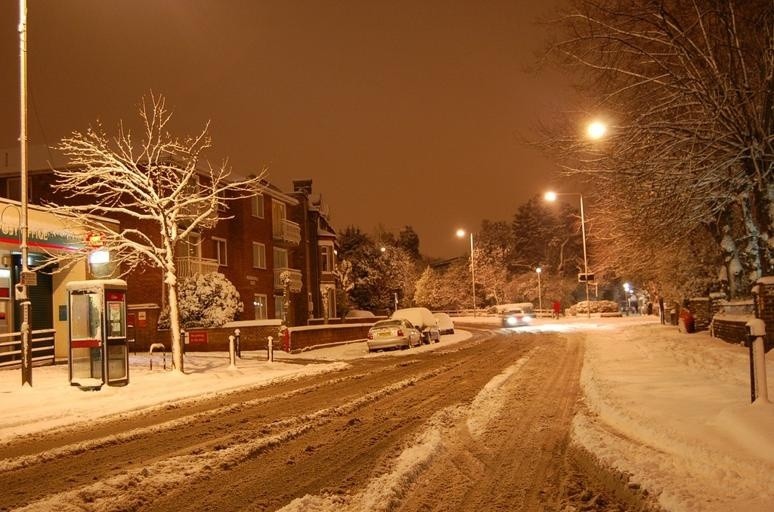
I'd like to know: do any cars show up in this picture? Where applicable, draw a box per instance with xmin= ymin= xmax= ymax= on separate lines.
xmin=366 ymin=308 xmax=454 ymax=353
xmin=501 ymin=308 xmax=530 ymax=327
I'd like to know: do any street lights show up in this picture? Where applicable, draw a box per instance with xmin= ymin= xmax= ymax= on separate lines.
xmin=535 ymin=267 xmax=543 ymax=320
xmin=623 ymin=283 xmax=633 ymax=317
xmin=541 ymin=190 xmax=591 ymax=318
xmin=455 ymin=227 xmax=476 ymax=318
xmin=380 ymin=246 xmax=397 ymax=310
